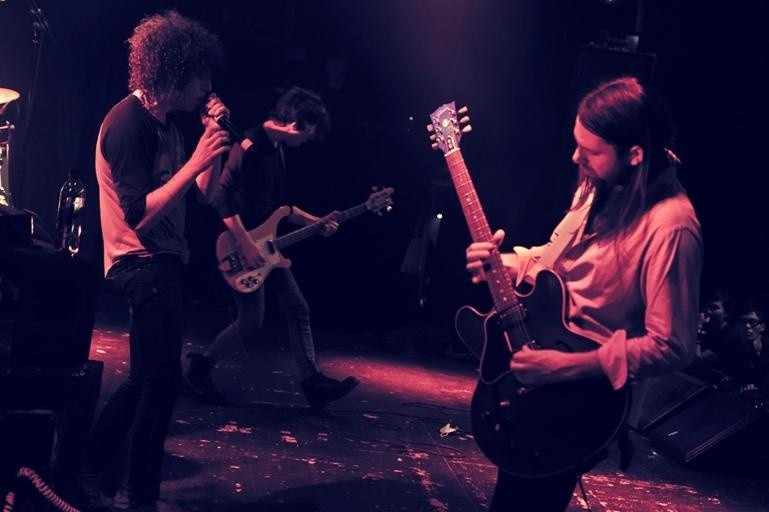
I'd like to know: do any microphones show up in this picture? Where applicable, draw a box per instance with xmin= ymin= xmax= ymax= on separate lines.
xmin=200 ymin=95 xmax=255 ymax=152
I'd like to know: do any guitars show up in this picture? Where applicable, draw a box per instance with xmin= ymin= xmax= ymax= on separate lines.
xmin=427 ymin=101 xmax=632 ymax=478
xmin=216 ymin=185 xmax=394 ymax=292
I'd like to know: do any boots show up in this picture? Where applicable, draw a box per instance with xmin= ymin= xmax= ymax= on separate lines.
xmin=182 ymin=352 xmax=227 ymax=404
xmin=299 ymin=370 xmax=359 ymax=411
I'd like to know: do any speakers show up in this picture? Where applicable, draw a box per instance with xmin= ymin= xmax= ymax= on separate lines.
xmin=624 ymin=362 xmax=714 ymax=436
xmin=650 ymin=387 xmax=769 ymax=470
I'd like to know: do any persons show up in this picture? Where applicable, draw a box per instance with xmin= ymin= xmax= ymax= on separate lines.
xmin=690 ymin=288 xmax=741 ymax=378
xmin=175 ymin=84 xmax=360 ymax=410
xmin=731 ymin=296 xmax=768 ymax=414
xmin=464 ymin=74 xmax=704 ymax=511
xmin=84 ymin=11 xmax=230 ymax=509
